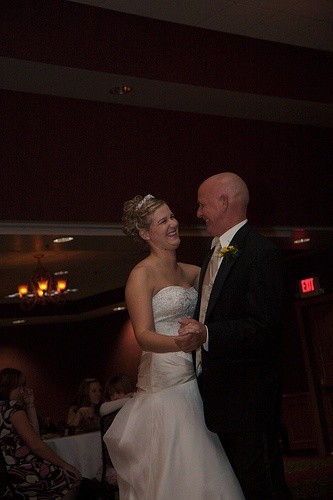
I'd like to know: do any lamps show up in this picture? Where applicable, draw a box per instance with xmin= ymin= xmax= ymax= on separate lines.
xmin=17 ymin=254 xmax=81 ymax=310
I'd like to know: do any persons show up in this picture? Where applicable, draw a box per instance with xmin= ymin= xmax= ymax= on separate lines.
xmin=67 ymin=378 xmax=105 ymax=433
xmin=0 ymin=368 xmax=82 ymax=500
xmin=99 ymin=375 xmax=134 ymax=486
xmin=102 ymin=194 xmax=245 ymax=500
xmin=23 ymin=387 xmax=40 ymax=437
xmin=179 ymin=172 xmax=292 ymax=500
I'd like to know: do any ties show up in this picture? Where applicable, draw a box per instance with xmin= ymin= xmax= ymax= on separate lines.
xmin=195 ymin=241 xmax=222 ymax=376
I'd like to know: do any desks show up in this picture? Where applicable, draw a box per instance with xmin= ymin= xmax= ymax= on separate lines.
xmin=41 ymin=430 xmax=103 ymax=481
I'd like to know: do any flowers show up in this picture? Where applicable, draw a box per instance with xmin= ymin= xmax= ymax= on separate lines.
xmin=216 ymin=240 xmax=239 ymax=263
